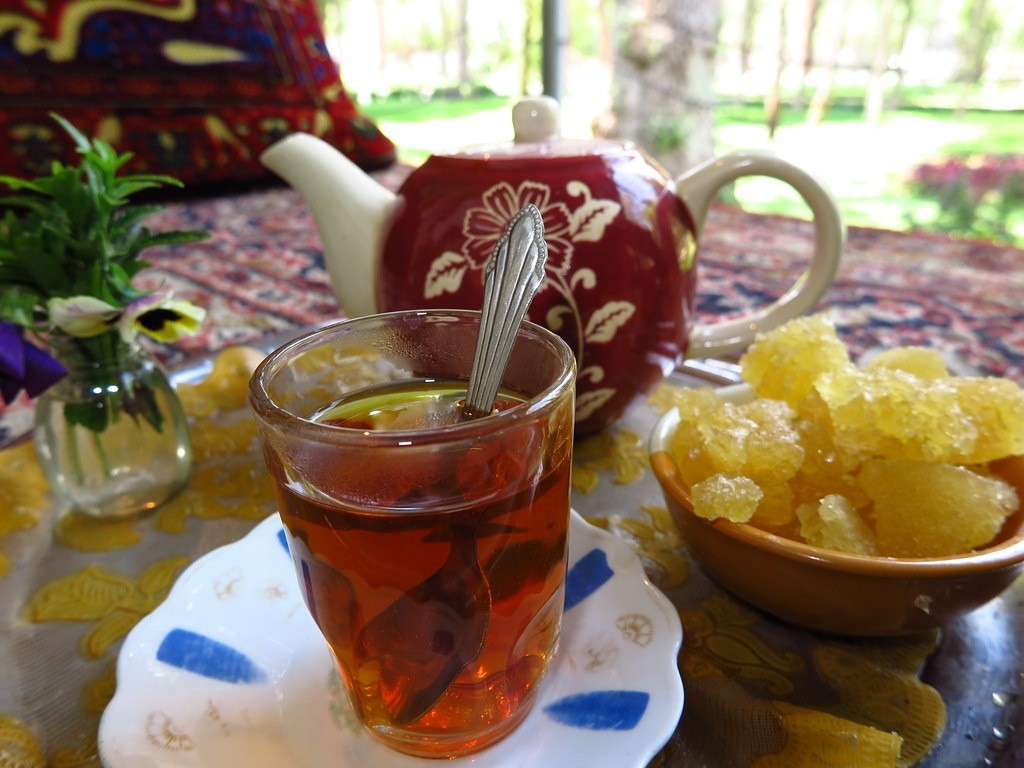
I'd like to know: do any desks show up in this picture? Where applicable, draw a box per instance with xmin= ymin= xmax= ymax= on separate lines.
xmin=0 ymin=317 xmax=1023 ymax=766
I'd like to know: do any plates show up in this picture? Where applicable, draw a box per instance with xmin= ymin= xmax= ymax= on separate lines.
xmin=96 ymin=504 xmax=687 ymax=767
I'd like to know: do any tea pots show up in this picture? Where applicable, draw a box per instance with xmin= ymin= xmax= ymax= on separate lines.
xmin=258 ymin=94 xmax=848 ymax=445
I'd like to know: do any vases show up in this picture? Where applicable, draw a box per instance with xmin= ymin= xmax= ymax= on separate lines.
xmin=32 ymin=326 xmax=193 ymax=520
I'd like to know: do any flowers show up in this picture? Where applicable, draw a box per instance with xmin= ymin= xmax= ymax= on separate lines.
xmin=0 ymin=111 xmax=213 ymax=485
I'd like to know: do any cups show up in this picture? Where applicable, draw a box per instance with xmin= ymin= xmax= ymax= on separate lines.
xmin=245 ymin=309 xmax=577 ymax=758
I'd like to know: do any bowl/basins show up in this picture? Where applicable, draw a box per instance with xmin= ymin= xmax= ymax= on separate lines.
xmin=646 ymin=382 xmax=1024 ymax=637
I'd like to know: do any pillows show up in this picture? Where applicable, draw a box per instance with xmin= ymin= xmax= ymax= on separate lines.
xmin=0 ymin=0 xmax=395 ymax=201
